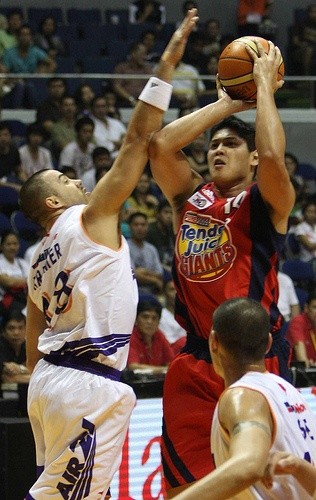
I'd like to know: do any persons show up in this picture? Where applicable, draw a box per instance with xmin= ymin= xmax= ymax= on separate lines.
xmin=0 ymin=0 xmax=316 ymax=500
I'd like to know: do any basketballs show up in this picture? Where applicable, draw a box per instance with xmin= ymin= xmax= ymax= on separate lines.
xmin=217 ymin=35 xmax=285 ymax=104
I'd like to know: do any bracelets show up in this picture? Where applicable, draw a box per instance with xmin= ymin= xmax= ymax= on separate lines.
xmin=137 ymin=77 xmax=174 ymax=114
